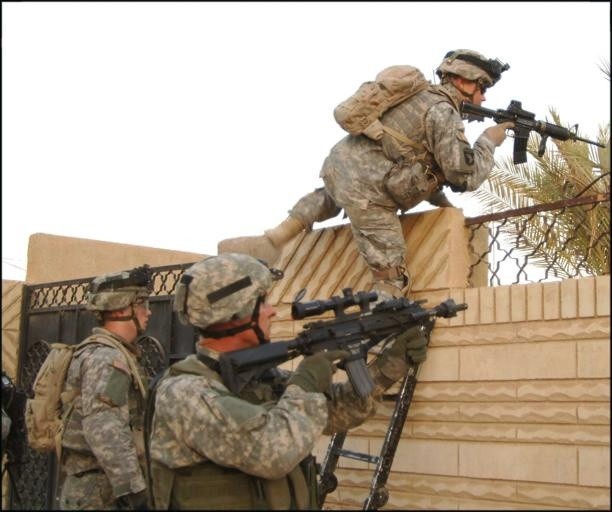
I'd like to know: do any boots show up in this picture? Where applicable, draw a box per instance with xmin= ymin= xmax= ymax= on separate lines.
xmin=263 ymin=216 xmax=306 ymax=248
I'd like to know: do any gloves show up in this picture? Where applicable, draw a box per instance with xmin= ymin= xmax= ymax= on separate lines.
xmin=367 ymin=325 xmax=430 ymax=388
xmin=286 ymin=348 xmax=353 ymax=395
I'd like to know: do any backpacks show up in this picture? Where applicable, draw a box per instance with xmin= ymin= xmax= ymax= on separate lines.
xmin=23 ymin=335 xmax=149 ymax=451
xmin=332 ymin=64 xmax=459 ymax=151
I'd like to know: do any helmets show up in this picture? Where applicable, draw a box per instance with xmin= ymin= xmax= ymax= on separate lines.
xmin=435 ymin=48 xmax=510 ymax=89
xmin=82 ymin=264 xmax=155 ymax=313
xmin=170 ymin=253 xmax=284 ymax=328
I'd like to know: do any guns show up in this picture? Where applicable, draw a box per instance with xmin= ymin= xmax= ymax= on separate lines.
xmin=460 ymin=100 xmax=607 ymax=164
xmin=218 ymin=288 xmax=469 ymax=397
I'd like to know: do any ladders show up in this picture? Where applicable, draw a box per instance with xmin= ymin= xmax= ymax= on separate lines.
xmin=318 ymin=314 xmax=436 ymax=512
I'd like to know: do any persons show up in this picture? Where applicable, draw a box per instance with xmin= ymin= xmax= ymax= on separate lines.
xmin=142 ymin=251 xmax=430 ymax=510
xmin=56 ymin=263 xmax=156 ymax=511
xmin=265 ymin=42 xmax=516 ymax=400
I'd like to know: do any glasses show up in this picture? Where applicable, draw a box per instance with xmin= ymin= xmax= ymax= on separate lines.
xmin=132 ymin=300 xmax=151 ymax=310
xmin=470 ymin=79 xmax=489 ymax=95
